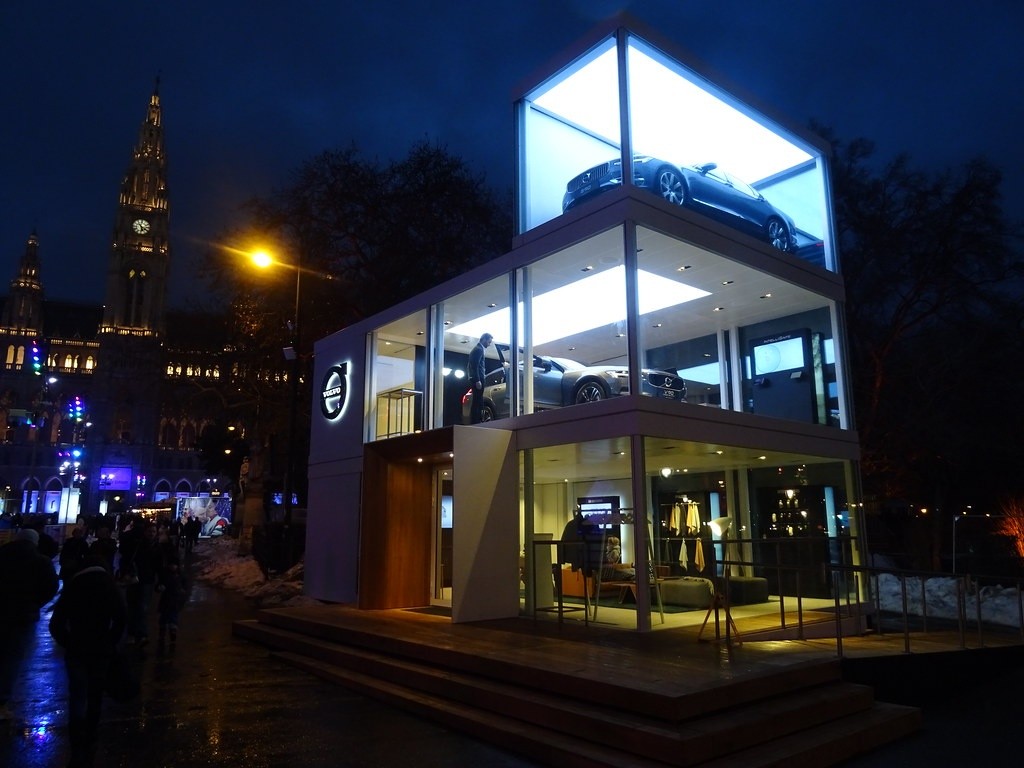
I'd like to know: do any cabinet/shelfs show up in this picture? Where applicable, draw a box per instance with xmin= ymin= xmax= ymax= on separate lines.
xmin=573 ymin=507 xmax=666 ymax=625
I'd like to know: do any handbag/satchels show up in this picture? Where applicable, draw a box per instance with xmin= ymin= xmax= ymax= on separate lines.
xmin=118 ymin=577 xmax=125 ymax=588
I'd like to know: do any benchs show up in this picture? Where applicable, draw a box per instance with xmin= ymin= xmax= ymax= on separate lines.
xmin=717 ymin=575 xmax=770 ymax=604
xmin=660 ymin=579 xmax=711 ymax=608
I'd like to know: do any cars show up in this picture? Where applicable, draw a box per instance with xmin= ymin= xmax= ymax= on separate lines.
xmin=560 ymin=153 xmax=800 ymax=254
xmin=459 ymin=343 xmax=689 ymax=425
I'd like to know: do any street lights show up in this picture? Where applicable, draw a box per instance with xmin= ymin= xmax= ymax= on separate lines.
xmin=60 ymin=449 xmax=82 ymax=542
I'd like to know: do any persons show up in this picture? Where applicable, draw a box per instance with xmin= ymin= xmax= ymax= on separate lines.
xmin=188 ymin=499 xmax=231 ymax=536
xmin=0 ymin=511 xmax=201 ymax=768
xmin=467 ymin=335 xmax=490 ymax=424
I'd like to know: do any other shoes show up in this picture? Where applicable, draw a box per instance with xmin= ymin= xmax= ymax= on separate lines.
xmin=160 ymin=635 xmax=165 ymax=638
xmin=171 ymin=632 xmax=176 ymax=641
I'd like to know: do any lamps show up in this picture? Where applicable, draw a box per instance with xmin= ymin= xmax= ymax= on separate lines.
xmin=700 ymin=516 xmax=743 ymax=647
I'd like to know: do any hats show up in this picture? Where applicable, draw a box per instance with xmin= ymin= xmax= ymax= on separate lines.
xmin=18 ymin=529 xmax=39 ymax=545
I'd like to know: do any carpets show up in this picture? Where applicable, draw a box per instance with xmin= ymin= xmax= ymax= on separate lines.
xmin=519 ymin=589 xmax=763 ymax=614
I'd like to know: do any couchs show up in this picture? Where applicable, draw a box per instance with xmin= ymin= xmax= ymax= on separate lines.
xmin=559 ymin=563 xmax=670 ymax=599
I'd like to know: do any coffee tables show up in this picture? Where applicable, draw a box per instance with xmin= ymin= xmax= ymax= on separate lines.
xmin=612 ymin=583 xmax=660 ymax=604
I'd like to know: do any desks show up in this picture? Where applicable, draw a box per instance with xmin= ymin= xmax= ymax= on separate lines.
xmin=376 ymin=388 xmax=424 ymax=438
xmin=656 ymin=565 xmax=671 ymax=578
